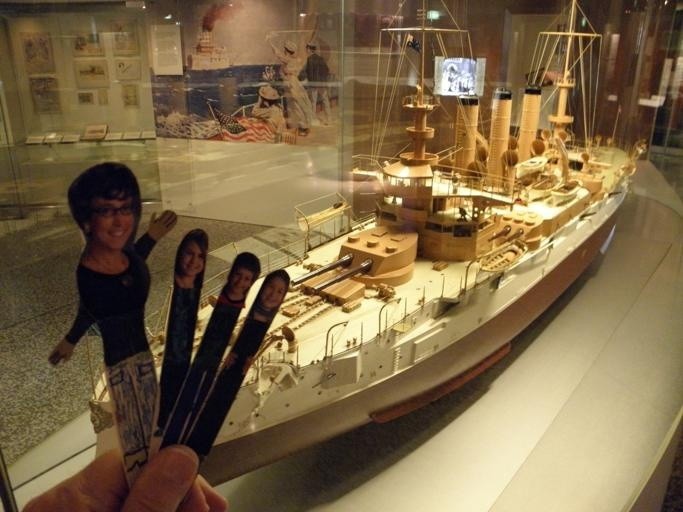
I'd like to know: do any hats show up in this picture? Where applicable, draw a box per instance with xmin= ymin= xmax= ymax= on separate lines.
xmin=284 ymin=39 xmax=318 ymax=52
xmin=258 ymin=85 xmax=280 ymax=100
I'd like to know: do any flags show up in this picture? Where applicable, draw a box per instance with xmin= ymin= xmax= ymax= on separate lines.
xmin=214 ymin=108 xmax=275 ymax=144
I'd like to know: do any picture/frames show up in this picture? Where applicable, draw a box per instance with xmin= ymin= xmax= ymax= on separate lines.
xmin=21 ymin=19 xmax=145 ymax=116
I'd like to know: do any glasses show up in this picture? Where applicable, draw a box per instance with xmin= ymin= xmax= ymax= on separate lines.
xmin=88 ymin=201 xmax=137 ymax=220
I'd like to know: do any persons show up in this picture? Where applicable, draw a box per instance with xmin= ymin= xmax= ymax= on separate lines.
xmin=298 ymin=40 xmax=333 ymax=126
xmin=158 ymin=250 xmax=263 ymax=454
xmin=252 ymin=87 xmax=286 ymax=142
xmin=188 ymin=268 xmax=292 ymax=459
xmin=20 ymin=443 xmax=230 ymax=512
xmin=269 ymin=37 xmax=313 ymax=137
xmin=150 ymin=225 xmax=207 ymax=437
xmin=45 ymin=163 xmax=177 ymax=484
